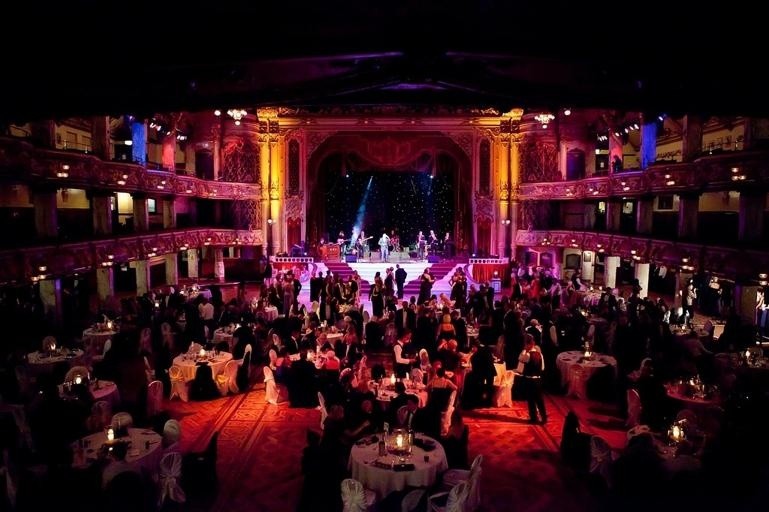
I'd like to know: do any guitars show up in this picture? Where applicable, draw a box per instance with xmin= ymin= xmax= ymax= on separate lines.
xmin=338 ymin=239 xmax=351 ymax=244
xmin=357 ymin=236 xmax=374 ymax=246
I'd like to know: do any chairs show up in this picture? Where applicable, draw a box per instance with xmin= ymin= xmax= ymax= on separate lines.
xmin=0 ymin=291 xmax=228 ymax=511
xmin=261 ymin=345 xmax=514 ymax=429
xmin=554 ymin=347 xmax=768 ymax=510
xmin=331 ymin=429 xmax=485 ymax=511
xmin=140 ymin=339 xmax=254 ymax=404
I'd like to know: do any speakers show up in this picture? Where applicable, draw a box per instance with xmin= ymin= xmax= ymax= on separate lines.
xmin=346 ymin=256 xmax=357 ymax=262
xmin=428 ymin=256 xmax=439 ymax=263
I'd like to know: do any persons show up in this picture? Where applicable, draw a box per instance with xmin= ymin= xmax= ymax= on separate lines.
xmin=316 ymin=237 xmax=325 ymax=256
xmin=262 ymin=269 xmax=302 ymax=313
xmin=389 ymin=231 xmax=396 ymax=239
xmin=448 ymin=258 xmax=768 ymax=435
xmin=417 ymin=267 xmax=436 ymax=304
xmin=1 ymin=413 xmax=191 ymax=511
xmin=416 ymin=229 xmax=451 ymax=261
xmin=337 ymin=231 xmax=372 ymax=260
xmin=378 ymin=233 xmax=390 ymax=262
xmin=304 ymin=395 xmax=469 ymax=512
xmin=612 ymin=155 xmax=622 ymax=174
xmin=310 ymin=269 xmax=361 ymax=304
xmin=374 ymin=264 xmax=407 ymax=298
xmin=106 ymin=281 xmax=268 ymax=401
xmin=268 ymin=294 xmax=455 ymax=411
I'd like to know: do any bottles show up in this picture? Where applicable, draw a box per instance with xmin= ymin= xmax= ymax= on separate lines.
xmin=680 ymin=421 xmax=685 ymax=438
xmin=379 ymin=439 xmax=386 ymax=456
xmin=408 ymin=427 xmax=414 ymax=445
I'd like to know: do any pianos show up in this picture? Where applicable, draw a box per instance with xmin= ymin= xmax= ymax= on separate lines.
xmin=320 ymin=244 xmax=341 ymax=258
